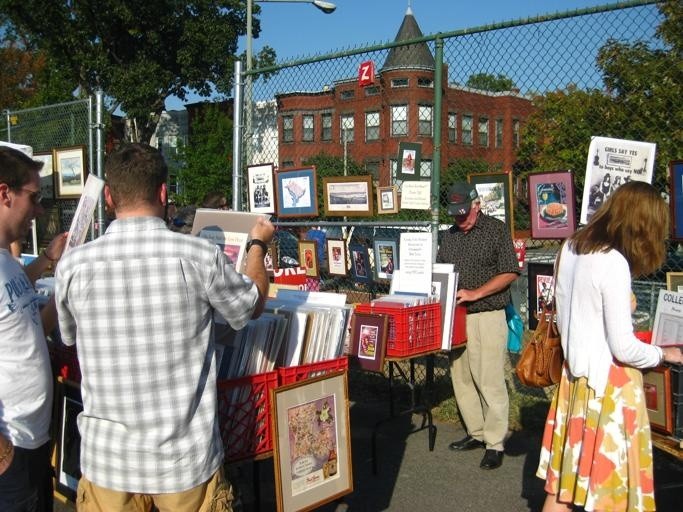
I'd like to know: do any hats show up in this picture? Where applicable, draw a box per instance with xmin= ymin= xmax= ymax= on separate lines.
xmin=448 ymin=183 xmax=478 ymax=215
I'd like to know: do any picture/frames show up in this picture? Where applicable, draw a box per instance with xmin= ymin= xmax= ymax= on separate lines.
xmin=348 ymin=313 xmax=388 ymax=373
xmin=54 ymin=382 xmax=82 ymax=502
xmin=245 ymin=163 xmax=276 ymax=215
xmin=395 ymin=141 xmax=421 ymax=181
xmin=326 ymin=237 xmax=347 ymax=276
xmin=377 ymin=186 xmax=398 ymax=214
xmin=264 ymin=245 xmax=277 ymax=272
xmin=274 ymin=165 xmax=320 ymax=217
xmin=526 ymin=169 xmax=578 ymax=240
xmin=468 ymin=171 xmax=515 ymax=241
xmin=669 ymin=160 xmax=683 ymax=241
xmin=52 ymin=145 xmax=86 ymax=199
xmin=666 ymin=272 xmax=683 ymax=294
xmin=32 ymin=151 xmax=58 ymax=208
xmin=297 ymin=240 xmax=320 ymax=278
xmin=271 ymin=369 xmax=353 ymax=512
xmin=350 ymin=246 xmax=372 ymax=283
xmin=373 ymin=237 xmax=400 ymax=282
xmin=322 ymin=176 xmax=374 ymax=217
xmin=528 ymin=263 xmax=557 ymax=331
xmin=641 ymin=366 xmax=673 ymax=436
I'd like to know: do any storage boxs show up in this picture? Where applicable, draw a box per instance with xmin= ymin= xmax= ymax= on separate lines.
xmin=216 ymin=370 xmax=278 ymax=462
xmin=353 ymin=304 xmax=440 ymax=358
xmin=280 ymin=354 xmax=349 ymax=384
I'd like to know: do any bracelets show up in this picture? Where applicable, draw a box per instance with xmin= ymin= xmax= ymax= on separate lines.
xmin=0 ymin=440 xmax=13 ymax=462
xmin=42 ymin=250 xmax=58 ymax=270
xmin=661 ymin=348 xmax=665 ymax=366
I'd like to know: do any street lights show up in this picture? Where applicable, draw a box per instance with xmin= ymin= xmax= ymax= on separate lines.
xmin=247 ymin=0 xmax=336 ymax=214
xmin=247 ymin=0 xmax=336 ymax=165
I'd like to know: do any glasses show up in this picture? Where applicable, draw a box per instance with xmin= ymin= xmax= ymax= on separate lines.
xmin=19 ymin=187 xmax=43 ymax=203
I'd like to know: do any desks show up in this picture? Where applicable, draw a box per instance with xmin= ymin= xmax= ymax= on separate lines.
xmin=224 ymin=450 xmax=273 ymax=512
xmin=371 ymin=343 xmax=467 ymax=474
xmin=652 ymin=430 xmax=683 ymax=462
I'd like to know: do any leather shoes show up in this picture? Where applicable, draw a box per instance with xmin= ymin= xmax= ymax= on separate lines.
xmin=450 ymin=434 xmax=481 ymax=451
xmin=480 ymin=450 xmax=502 ymax=469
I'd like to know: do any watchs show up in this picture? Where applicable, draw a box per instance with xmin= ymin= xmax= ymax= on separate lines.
xmin=246 ymin=239 xmax=268 ymax=256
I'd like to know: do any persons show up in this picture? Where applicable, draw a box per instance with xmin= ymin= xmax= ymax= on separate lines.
xmin=534 ymin=180 xmax=682 ymax=512
xmin=26 ymin=232 xmax=68 ymax=292
xmin=305 ymin=227 xmax=325 ymax=263
xmin=364 ymin=238 xmax=375 ymax=270
xmin=435 ymin=182 xmax=521 ymax=471
xmin=612 ymin=176 xmax=621 ymax=191
xmin=167 ymin=198 xmax=177 ymax=218
xmin=540 ymin=282 xmax=553 ymax=306
xmin=589 ymin=185 xmax=600 ymax=208
xmin=276 ymin=220 xmax=309 ymax=268
xmin=9 ymin=241 xmax=23 ymax=258
xmin=625 ymin=176 xmax=633 ymax=184
xmin=386 ymin=258 xmax=393 ymax=273
xmin=54 ymin=142 xmax=275 ymax=512
xmin=202 ymin=191 xmax=230 ymax=210
xmin=594 ymin=184 xmax=604 ymax=208
xmin=355 ymin=253 xmax=365 ymax=276
xmin=0 ymin=146 xmax=59 ymax=512
xmin=600 ymin=173 xmax=612 ymax=202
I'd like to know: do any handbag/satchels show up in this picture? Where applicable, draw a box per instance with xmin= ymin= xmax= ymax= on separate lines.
xmin=516 ymin=239 xmax=564 ymax=387
